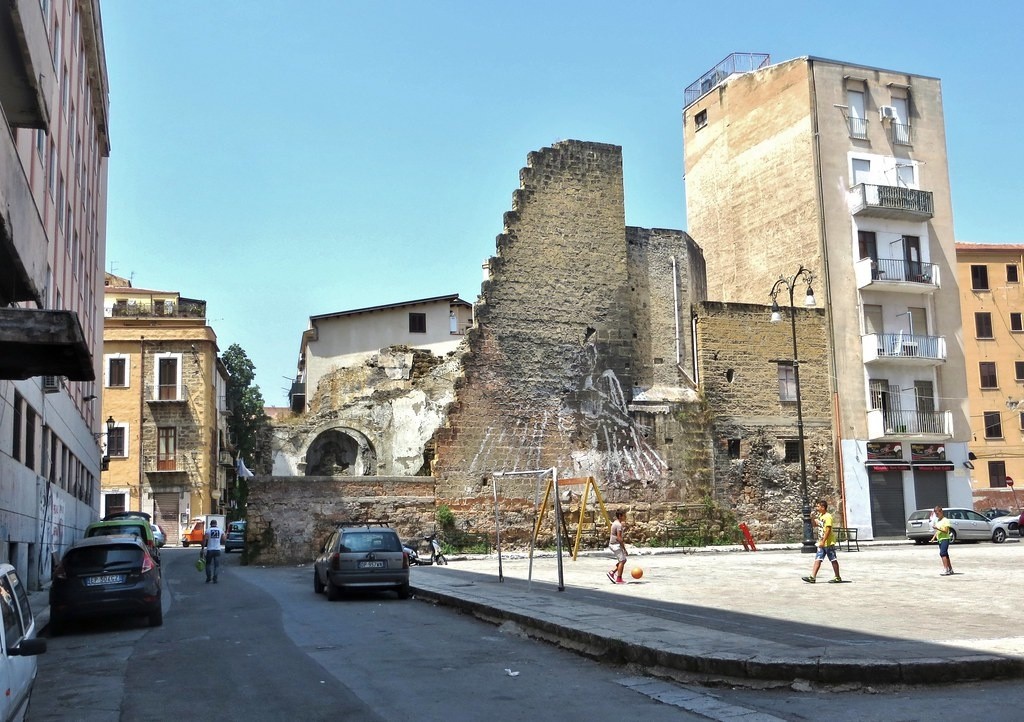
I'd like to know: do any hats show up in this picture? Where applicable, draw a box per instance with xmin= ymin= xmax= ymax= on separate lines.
xmin=210 ymin=520 xmax=218 ymax=525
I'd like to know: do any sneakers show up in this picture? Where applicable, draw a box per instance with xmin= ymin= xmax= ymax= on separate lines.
xmin=949 ymin=569 xmax=953 ymax=573
xmin=802 ymin=575 xmax=815 ymax=583
xmin=828 ymin=577 xmax=842 ymax=582
xmin=940 ymin=569 xmax=950 ymax=574
xmin=616 ymin=579 xmax=626 ymax=583
xmin=607 ymin=572 xmax=616 ymax=583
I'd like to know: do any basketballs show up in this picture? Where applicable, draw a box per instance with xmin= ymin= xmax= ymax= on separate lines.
xmin=631 ymin=567 xmax=643 ymax=579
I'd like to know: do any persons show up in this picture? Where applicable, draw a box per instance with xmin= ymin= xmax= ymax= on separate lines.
xmin=200 ymin=520 xmax=226 ymax=584
xmin=931 ymin=506 xmax=954 ymax=575
xmin=606 ymin=507 xmax=628 ymax=584
xmin=802 ymin=500 xmax=843 ymax=583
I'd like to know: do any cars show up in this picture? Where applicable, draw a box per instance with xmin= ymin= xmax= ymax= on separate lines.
xmin=985 ymin=509 xmax=1022 ymax=537
xmin=0 ymin=563 xmax=46 ymax=722
xmin=48 ymin=511 xmax=167 ymax=627
xmin=225 ymin=518 xmax=247 ymax=553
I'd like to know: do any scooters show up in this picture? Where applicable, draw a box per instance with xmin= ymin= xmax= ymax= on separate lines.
xmin=402 ymin=532 xmax=447 ymax=566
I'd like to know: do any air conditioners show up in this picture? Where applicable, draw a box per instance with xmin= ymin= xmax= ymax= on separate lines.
xmin=41 ymin=376 xmax=61 ymax=393
xmin=879 ymin=105 xmax=897 ymax=120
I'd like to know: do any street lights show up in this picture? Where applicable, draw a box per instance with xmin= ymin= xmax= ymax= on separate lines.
xmin=768 ymin=264 xmax=817 ymax=553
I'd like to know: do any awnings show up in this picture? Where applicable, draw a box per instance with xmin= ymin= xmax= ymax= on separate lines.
xmin=866 ymin=460 xmax=954 ymax=471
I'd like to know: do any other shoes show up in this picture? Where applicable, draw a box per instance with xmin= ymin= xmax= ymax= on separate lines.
xmin=206 ymin=578 xmax=210 ymax=582
xmin=213 ymin=577 xmax=217 ymax=584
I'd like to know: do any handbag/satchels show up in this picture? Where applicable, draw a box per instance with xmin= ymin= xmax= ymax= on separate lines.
xmin=195 ymin=553 xmax=206 ymax=572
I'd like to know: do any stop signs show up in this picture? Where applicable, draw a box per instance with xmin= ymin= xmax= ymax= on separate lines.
xmin=1005 ymin=476 xmax=1013 ymax=486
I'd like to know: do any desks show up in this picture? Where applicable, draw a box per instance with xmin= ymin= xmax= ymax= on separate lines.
xmin=902 ymin=342 xmax=918 ymax=357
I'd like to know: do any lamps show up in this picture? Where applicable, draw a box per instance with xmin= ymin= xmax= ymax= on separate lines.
xmin=94 ymin=415 xmax=116 ymax=444
xmin=83 ymin=395 xmax=98 ymax=402
xmin=963 ymin=461 xmax=974 ymax=469
xmin=191 ymin=449 xmax=198 ymax=464
xmin=969 ymin=451 xmax=977 ymax=460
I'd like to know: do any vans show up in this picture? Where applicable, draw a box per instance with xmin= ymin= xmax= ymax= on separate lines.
xmin=906 ymin=507 xmax=1009 ymax=545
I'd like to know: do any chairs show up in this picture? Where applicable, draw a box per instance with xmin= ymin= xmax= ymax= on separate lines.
xmin=368 ymin=538 xmax=385 ymax=551
xmin=871 ymin=261 xmax=885 ymax=280
xmin=955 ymin=512 xmax=962 ymax=519
xmin=877 ymin=341 xmax=884 ymax=355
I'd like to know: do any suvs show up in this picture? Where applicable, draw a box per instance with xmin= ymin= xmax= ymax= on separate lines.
xmin=314 ymin=519 xmax=410 ymax=600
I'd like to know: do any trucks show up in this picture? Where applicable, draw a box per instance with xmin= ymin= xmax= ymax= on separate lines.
xmin=181 ymin=514 xmax=226 ymax=547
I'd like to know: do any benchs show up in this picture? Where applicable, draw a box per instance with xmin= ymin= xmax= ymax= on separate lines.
xmin=667 ymin=526 xmax=701 ymax=548
xmin=832 ymin=528 xmax=860 ymax=552
